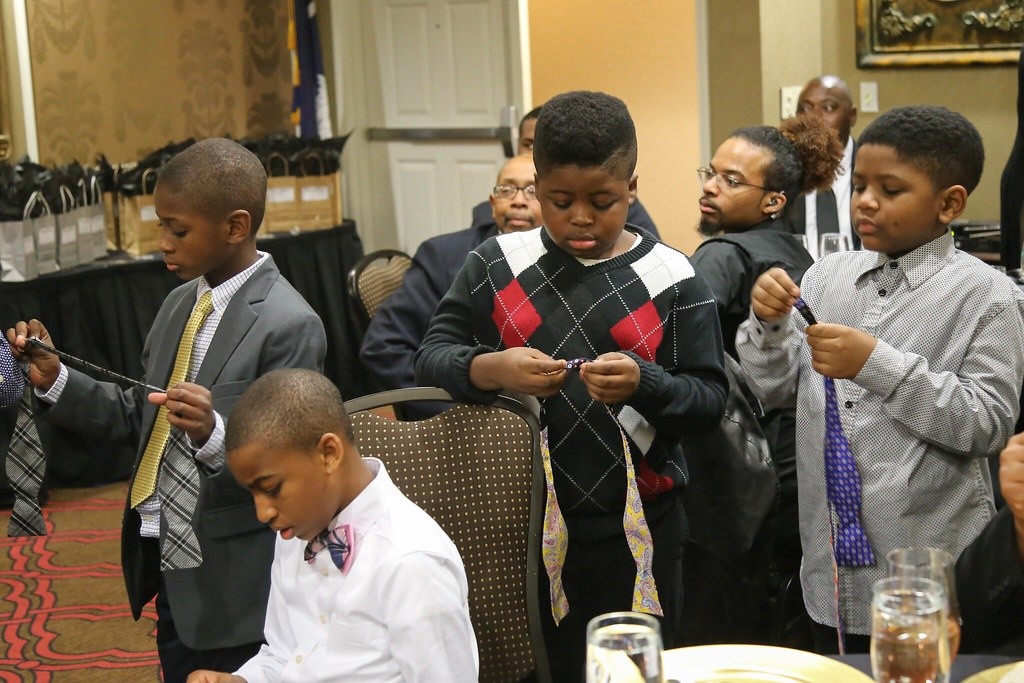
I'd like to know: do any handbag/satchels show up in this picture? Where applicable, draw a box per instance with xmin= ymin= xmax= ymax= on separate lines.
xmin=686 ymin=233 xmax=778 ymax=570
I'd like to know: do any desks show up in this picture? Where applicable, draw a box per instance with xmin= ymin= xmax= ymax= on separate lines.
xmin=0 ymin=217 xmax=379 ymax=514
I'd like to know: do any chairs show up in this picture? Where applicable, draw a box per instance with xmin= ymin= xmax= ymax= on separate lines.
xmin=348 ymin=248 xmax=415 ymax=327
xmin=338 ymin=388 xmax=551 ymax=683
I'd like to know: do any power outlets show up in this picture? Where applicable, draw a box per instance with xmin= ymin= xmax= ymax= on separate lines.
xmin=861 ymin=82 xmax=880 ymax=115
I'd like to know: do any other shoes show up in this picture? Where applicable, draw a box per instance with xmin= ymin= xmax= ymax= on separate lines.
xmin=0 ymin=124 xmax=355 ymax=222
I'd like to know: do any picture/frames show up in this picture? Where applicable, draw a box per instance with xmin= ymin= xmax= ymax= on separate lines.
xmin=854 ymin=0 xmax=1024 ymax=69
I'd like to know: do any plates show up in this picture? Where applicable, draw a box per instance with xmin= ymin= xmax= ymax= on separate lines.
xmin=664 ymin=644 xmax=875 ymax=683
xmin=960 ymin=661 xmax=1024 ymax=683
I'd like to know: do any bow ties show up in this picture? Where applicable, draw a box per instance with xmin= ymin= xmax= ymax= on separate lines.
xmin=303 ymin=522 xmax=354 ymax=578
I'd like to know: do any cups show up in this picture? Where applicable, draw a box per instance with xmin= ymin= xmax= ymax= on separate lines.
xmin=586 ymin=612 xmax=664 ymax=683
xmin=871 ymin=577 xmax=950 ymax=683
xmin=887 ymin=547 xmax=960 ymax=663
xmin=792 ymin=235 xmax=809 ymax=251
xmin=821 ymin=233 xmax=849 ymax=259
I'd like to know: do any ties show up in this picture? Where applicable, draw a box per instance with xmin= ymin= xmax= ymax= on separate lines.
xmin=785 ymin=293 xmax=876 ymax=568
xmin=818 ymin=183 xmax=840 ymax=263
xmin=128 ymin=289 xmax=214 ymax=509
xmin=538 ymin=356 xmax=665 ymax=627
xmin=5 ymin=337 xmax=203 ymax=570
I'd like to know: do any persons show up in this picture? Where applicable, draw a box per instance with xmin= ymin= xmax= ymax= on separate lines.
xmin=779 ymin=76 xmax=863 ymax=262
xmin=413 ymin=90 xmax=731 ymax=683
xmin=472 ymin=105 xmax=661 ymax=242
xmin=187 ymin=367 xmax=479 ymax=682
xmin=954 ymin=431 xmax=1024 ymax=649
xmin=735 ymin=104 xmax=1024 ymax=658
xmin=359 ymin=152 xmax=545 ymax=425
xmin=686 ymin=112 xmax=817 ymax=654
xmin=6 ymin=136 xmax=327 ymax=683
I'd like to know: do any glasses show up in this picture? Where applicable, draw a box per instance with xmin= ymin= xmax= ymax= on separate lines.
xmin=495 ymin=182 xmax=538 ymax=201
xmin=697 ymin=165 xmax=769 ymax=196
